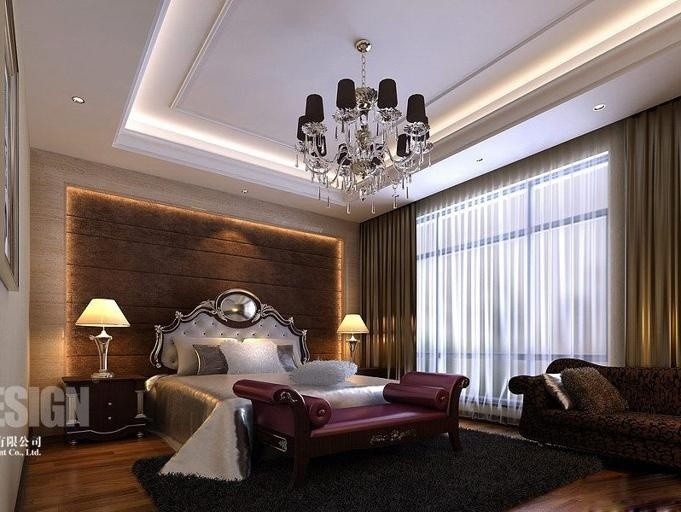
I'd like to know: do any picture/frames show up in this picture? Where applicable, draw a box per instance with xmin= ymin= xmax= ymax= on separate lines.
xmin=0 ymin=0 xmax=19 ymax=294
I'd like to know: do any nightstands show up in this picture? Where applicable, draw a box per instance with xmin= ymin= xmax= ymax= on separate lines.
xmin=355 ymin=368 xmax=384 ymax=379
xmin=62 ymin=375 xmax=146 ymax=446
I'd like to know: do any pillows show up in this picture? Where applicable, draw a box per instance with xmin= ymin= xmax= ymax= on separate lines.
xmin=288 ymin=359 xmax=358 ymax=386
xmin=541 ymin=373 xmax=572 ymax=411
xmin=560 ymin=366 xmax=629 ymax=414
xmin=220 ymin=339 xmax=285 ymax=375
xmin=189 ymin=343 xmax=228 ymax=376
xmin=242 ymin=338 xmax=303 ymax=368
xmin=171 ymin=335 xmax=237 ymax=377
xmin=275 ymin=344 xmax=298 ymax=372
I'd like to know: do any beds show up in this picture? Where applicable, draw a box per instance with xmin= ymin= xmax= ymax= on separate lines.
xmin=143 ymin=285 xmax=400 ymax=460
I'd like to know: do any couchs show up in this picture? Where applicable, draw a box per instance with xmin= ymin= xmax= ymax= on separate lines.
xmin=508 ymin=357 xmax=680 ymax=470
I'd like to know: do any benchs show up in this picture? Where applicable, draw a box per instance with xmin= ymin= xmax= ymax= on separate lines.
xmin=231 ymin=371 xmax=471 ymax=492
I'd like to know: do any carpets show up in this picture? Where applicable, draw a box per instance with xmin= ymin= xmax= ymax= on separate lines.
xmin=129 ymin=426 xmax=604 ymax=511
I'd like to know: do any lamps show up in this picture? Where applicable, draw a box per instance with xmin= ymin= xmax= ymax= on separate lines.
xmin=74 ymin=298 xmax=132 ymax=380
xmin=295 ymin=37 xmax=433 ymax=216
xmin=336 ymin=313 xmax=369 ymax=364
